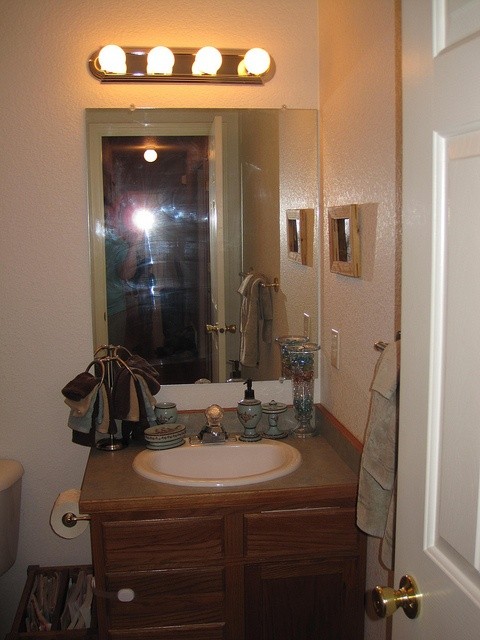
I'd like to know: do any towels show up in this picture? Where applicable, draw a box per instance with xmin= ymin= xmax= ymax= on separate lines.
xmin=114 ymin=355 xmax=160 ymax=439
xmin=61 ymin=372 xmax=116 ymax=448
xmin=238 ymin=274 xmax=274 ymax=368
xmin=355 ymin=343 xmax=400 ymax=569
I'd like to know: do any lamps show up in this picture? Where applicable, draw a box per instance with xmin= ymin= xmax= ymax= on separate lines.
xmin=98 ymin=45 xmax=127 ymax=74
xmin=193 ymin=47 xmax=223 ymax=73
xmin=144 ymin=146 xmax=158 ymax=162
xmin=245 ymin=48 xmax=269 ymax=76
xmin=146 ymin=46 xmax=175 ymax=76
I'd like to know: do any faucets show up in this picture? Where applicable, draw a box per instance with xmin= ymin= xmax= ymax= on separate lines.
xmin=196 ymin=405 xmax=228 ymax=442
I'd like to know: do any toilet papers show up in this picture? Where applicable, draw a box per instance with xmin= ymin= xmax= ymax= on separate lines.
xmin=49 ymin=489 xmax=89 ymax=540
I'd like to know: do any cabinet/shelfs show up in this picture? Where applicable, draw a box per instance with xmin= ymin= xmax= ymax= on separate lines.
xmin=79 ymin=489 xmax=369 ymax=640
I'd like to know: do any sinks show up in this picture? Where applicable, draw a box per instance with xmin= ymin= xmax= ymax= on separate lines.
xmin=133 ymin=437 xmax=302 ymax=487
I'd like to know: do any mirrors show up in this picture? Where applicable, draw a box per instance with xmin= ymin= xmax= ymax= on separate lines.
xmin=85 ymin=108 xmax=320 ymax=384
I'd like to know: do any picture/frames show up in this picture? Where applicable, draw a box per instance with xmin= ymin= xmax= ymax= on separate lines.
xmin=328 ymin=203 xmax=362 ymax=278
xmin=286 ymin=210 xmax=307 ymax=264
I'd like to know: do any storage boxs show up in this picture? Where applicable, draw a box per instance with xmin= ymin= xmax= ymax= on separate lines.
xmin=10 ymin=564 xmax=96 ymax=640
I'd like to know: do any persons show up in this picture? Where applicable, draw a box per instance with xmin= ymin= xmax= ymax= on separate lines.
xmin=104 ymin=202 xmax=138 ymax=359
